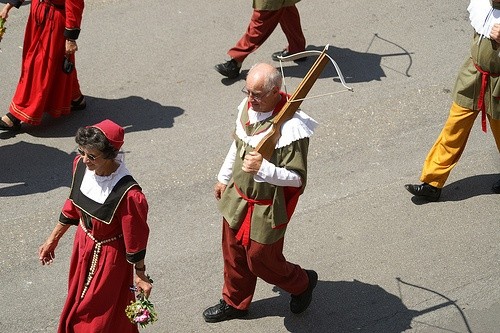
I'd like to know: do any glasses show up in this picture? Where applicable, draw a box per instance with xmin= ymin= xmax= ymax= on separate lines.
xmin=242 ymin=85 xmax=273 ymax=99
xmin=77 ymin=146 xmax=105 ymax=160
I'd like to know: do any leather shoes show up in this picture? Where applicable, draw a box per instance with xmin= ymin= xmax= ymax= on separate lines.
xmin=405 ymin=183 xmax=441 ymax=200
xmin=289 ymin=270 xmax=318 ymax=313
xmin=203 ymin=300 xmax=249 ymax=323
xmin=272 ymin=51 xmax=306 ymax=61
xmin=214 ymin=59 xmax=240 ymax=78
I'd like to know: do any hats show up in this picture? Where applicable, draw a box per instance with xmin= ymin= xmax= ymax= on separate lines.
xmin=89 ymin=119 xmax=125 ymax=148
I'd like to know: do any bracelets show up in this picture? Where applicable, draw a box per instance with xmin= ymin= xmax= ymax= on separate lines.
xmin=134 ymin=265 xmax=145 ymax=272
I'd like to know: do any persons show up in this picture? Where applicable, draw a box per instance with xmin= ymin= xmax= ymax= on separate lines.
xmin=213 ymin=0 xmax=306 ymax=77
xmin=404 ymin=0 xmax=500 ymax=201
xmin=40 ymin=119 xmax=152 ymax=333
xmin=202 ymin=63 xmax=318 ymax=323
xmin=0 ymin=0 xmax=87 ymax=130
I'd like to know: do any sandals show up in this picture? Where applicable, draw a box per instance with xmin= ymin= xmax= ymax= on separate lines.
xmin=0 ymin=113 xmax=22 ymax=131
xmin=70 ymin=95 xmax=87 ymax=109
